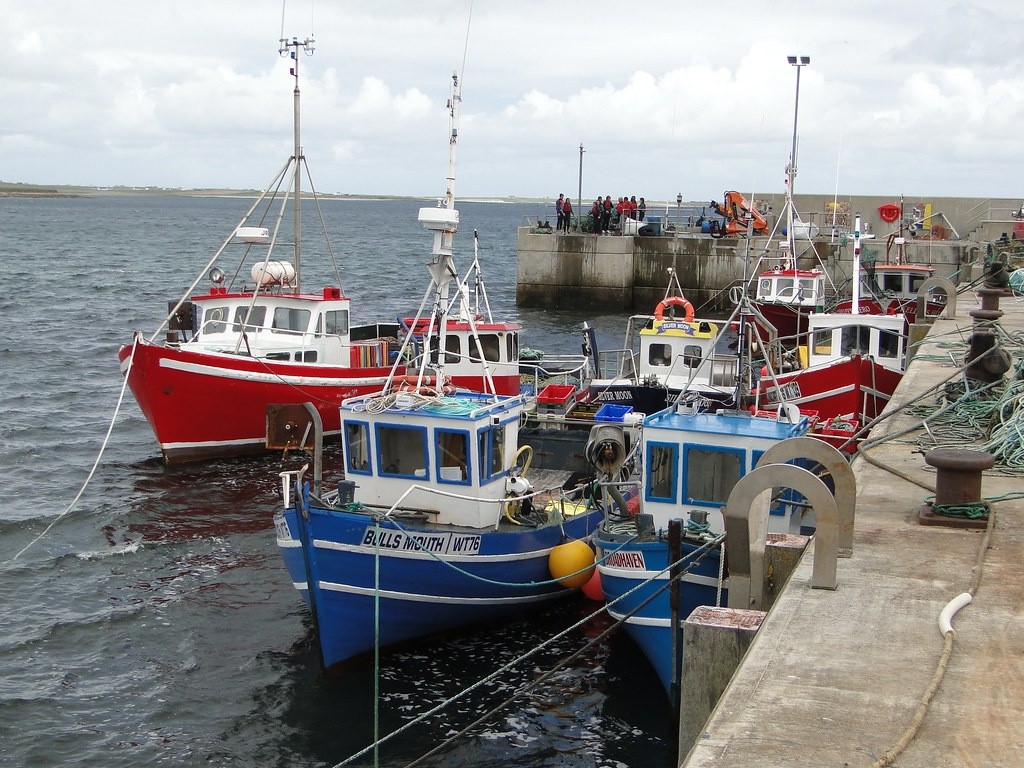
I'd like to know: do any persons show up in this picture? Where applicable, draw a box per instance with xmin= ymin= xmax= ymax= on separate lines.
xmin=588 ymin=196 xmax=604 ymax=238
xmin=638 ymin=197 xmax=646 ymax=222
xmin=561 ymin=198 xmax=578 ymax=236
xmin=555 ymin=193 xmax=565 ymax=230
xmin=603 ymin=195 xmax=614 ymax=234
xmin=615 ymin=196 xmax=637 ymax=228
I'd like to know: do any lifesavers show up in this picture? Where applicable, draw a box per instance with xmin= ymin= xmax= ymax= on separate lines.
xmin=392 ymin=375 xmax=456 ymax=396
xmin=438 ymin=314 xmax=484 ymax=325
xmin=879 ymin=208 xmax=899 ymax=222
xmin=654 ymin=296 xmax=695 ymax=322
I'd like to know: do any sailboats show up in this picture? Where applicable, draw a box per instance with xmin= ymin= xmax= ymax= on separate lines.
xmin=270 ymin=73 xmax=670 ymax=672
xmin=589 ymin=140 xmax=937 ymax=703
xmin=117 ymin=35 xmax=521 ymax=467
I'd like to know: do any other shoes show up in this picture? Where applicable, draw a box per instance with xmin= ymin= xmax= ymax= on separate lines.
xmin=556 ymin=230 xmax=562 ymax=234
xmin=602 ymin=230 xmax=611 ymax=234
xmin=568 ymin=230 xmax=570 ymax=234
xmin=563 ymin=232 xmax=566 ymax=235
xmin=592 ymin=233 xmax=598 ymax=236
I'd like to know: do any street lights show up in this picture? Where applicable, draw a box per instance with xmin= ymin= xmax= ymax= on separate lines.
xmin=784 ymin=54 xmax=811 ymax=199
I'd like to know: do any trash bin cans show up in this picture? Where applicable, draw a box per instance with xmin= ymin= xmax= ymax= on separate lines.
xmin=647 ymin=215 xmax=661 ymax=235
